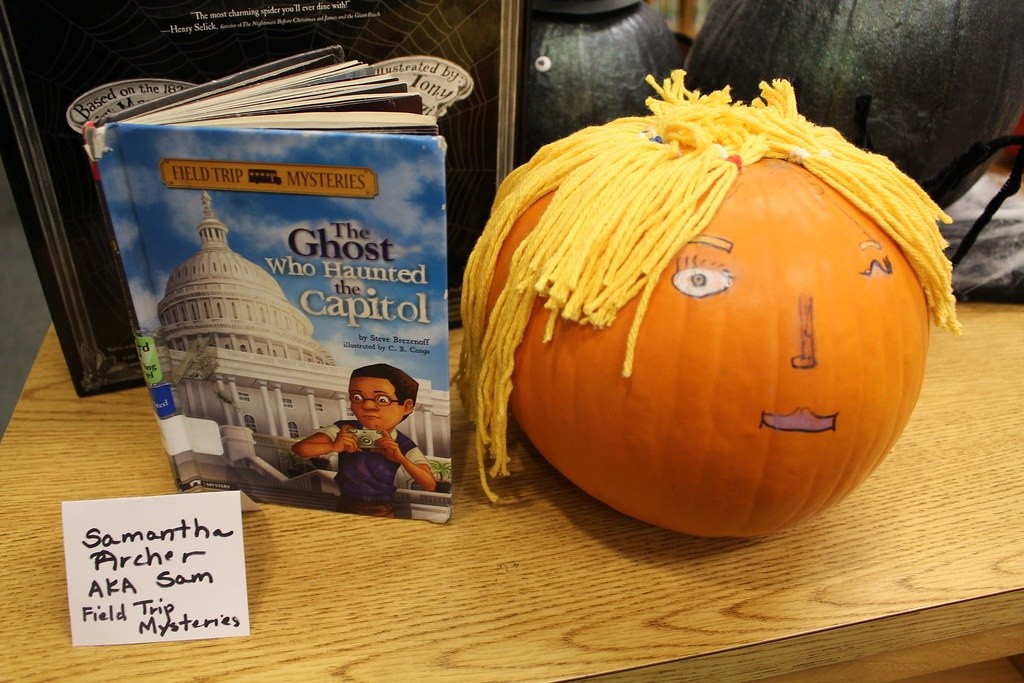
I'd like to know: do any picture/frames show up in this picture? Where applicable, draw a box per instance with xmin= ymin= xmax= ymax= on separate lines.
xmin=0 ymin=0 xmax=521 ymax=398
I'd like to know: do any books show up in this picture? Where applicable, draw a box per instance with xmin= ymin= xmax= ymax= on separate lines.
xmin=83 ymin=42 xmax=452 ymax=527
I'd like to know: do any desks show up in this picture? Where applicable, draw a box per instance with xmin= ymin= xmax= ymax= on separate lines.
xmin=0 ymin=155 xmax=1024 ymax=683
